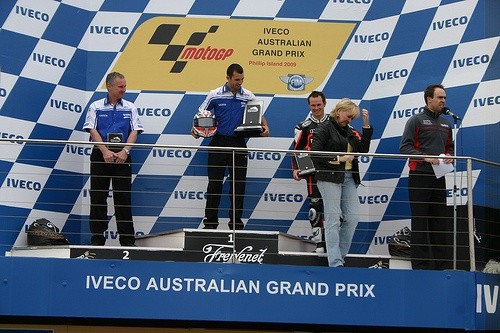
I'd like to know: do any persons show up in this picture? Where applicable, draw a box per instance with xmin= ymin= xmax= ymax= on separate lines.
xmin=399 ymin=84 xmax=454 ymax=269
xmin=310 ymin=98 xmax=373 ymax=268
xmin=292 ymin=91 xmax=362 ymax=253
xmin=82 ymin=72 xmax=144 ymax=246
xmin=191 ymin=63 xmax=269 ymax=230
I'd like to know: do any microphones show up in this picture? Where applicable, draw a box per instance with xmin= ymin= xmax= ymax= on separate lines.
xmin=441 ymin=107 xmax=459 ymax=120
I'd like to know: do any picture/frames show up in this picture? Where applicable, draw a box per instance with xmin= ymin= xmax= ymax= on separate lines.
xmin=294 ymin=152 xmax=315 ymax=171
xmin=107 ymin=133 xmax=123 ymax=148
xmin=242 ymin=100 xmax=263 ymax=125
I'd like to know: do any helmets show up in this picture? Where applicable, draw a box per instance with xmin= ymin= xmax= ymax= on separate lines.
xmin=27 ymin=218 xmax=59 ymax=246
xmin=192 ymin=110 xmax=218 ymax=138
xmin=388 ymin=227 xmax=411 ymax=258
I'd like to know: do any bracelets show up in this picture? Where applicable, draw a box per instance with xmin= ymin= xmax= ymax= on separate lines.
xmin=123 ymin=148 xmax=130 ymax=154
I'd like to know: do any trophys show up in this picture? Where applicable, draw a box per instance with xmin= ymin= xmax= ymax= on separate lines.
xmin=294 ymin=152 xmax=317 ymax=178
xmin=107 ymin=133 xmax=124 ymax=162
xmin=234 ymin=100 xmax=266 ymax=137
